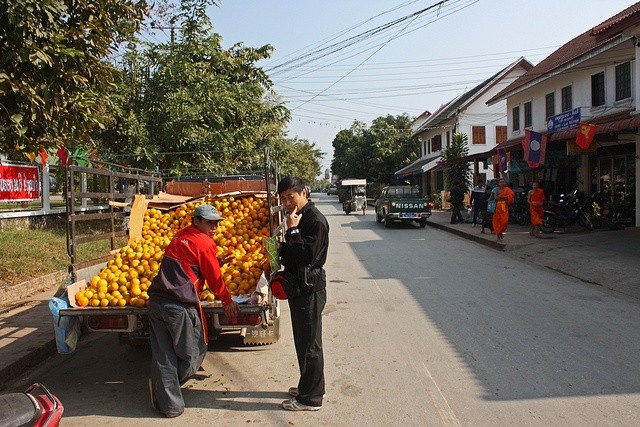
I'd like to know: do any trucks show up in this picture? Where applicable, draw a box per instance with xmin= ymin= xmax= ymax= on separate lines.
xmin=57 ymin=146 xmax=286 ymax=345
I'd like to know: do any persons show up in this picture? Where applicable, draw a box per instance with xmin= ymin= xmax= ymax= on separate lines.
xmin=528 ymin=181 xmax=545 ymax=237
xmin=450 ymin=181 xmax=463 ymax=224
xmin=489 ymin=178 xmax=515 ymax=239
xmin=146 ymin=203 xmax=241 ymax=418
xmin=480 ymin=185 xmax=495 ymax=233
xmin=274 ymin=176 xmax=329 ymax=411
xmin=470 ymin=179 xmax=485 ymax=226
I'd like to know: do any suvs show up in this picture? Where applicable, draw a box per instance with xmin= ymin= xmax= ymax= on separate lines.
xmin=327 ymin=185 xmax=338 ymax=195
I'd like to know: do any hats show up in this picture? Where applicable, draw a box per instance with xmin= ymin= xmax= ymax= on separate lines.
xmin=193 ymin=204 xmax=225 ymax=220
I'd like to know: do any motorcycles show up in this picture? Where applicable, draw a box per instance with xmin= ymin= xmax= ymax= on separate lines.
xmin=512 ymin=184 xmax=636 ymax=233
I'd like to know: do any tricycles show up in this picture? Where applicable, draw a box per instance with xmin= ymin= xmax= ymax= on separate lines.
xmin=341 ymin=179 xmax=367 ymax=215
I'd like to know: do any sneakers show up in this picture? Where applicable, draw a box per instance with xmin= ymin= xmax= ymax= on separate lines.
xmin=147 ymin=378 xmax=160 ymax=411
xmin=282 ymin=397 xmax=323 ymax=411
xmin=289 ymin=387 xmax=326 ymax=399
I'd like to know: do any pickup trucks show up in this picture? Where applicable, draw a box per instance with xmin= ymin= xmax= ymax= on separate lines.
xmin=375 ymin=186 xmax=432 ymax=228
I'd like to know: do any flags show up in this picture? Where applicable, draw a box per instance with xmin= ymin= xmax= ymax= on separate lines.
xmin=576 ymin=123 xmax=596 ymax=149
xmin=524 ymin=128 xmax=547 ymax=163
xmin=521 ymin=139 xmax=541 ymax=168
xmin=497 ymin=147 xmax=510 ymax=172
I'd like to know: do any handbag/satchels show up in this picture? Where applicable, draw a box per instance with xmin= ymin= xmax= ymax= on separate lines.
xmin=270 ymin=270 xmax=301 ymax=300
xmin=486 ymin=186 xmax=498 ymax=214
